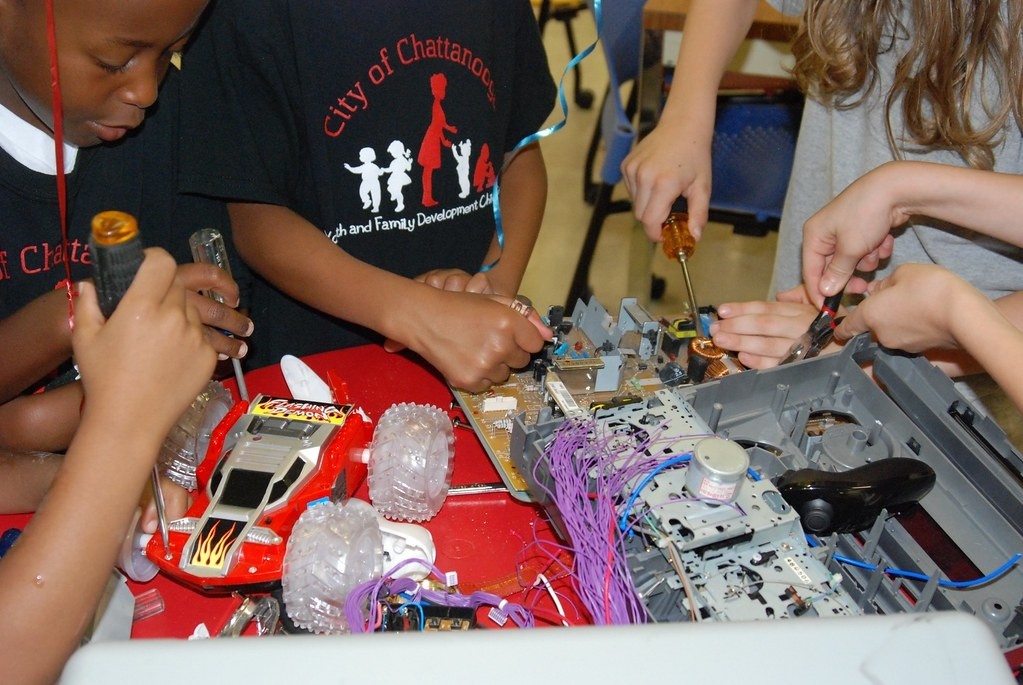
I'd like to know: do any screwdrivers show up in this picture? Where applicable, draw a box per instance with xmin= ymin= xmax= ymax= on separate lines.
xmin=186 ymin=227 xmax=248 ymax=417
xmin=83 ymin=208 xmax=178 ymax=564
xmin=653 ymin=196 xmax=707 ymax=342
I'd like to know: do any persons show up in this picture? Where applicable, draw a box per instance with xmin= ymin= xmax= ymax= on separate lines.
xmin=621 ymin=0 xmax=1022 ymax=382
xmin=800 ymin=157 xmax=1022 ymax=416
xmin=0 ymin=0 xmax=254 ymax=659
xmin=170 ymin=1 xmax=569 ymax=399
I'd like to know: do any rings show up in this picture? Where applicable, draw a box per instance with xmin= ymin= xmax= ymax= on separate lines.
xmin=509 ymin=300 xmax=533 ymax=317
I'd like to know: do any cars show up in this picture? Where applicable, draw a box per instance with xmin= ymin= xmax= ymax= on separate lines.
xmin=142 ymin=392 xmax=371 ymax=592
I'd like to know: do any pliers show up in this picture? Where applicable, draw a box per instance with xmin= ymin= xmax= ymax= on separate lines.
xmin=771 ymin=286 xmax=858 ymax=371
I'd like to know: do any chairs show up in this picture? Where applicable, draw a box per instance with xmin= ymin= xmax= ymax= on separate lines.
xmin=555 ymin=1 xmax=805 ymax=331
